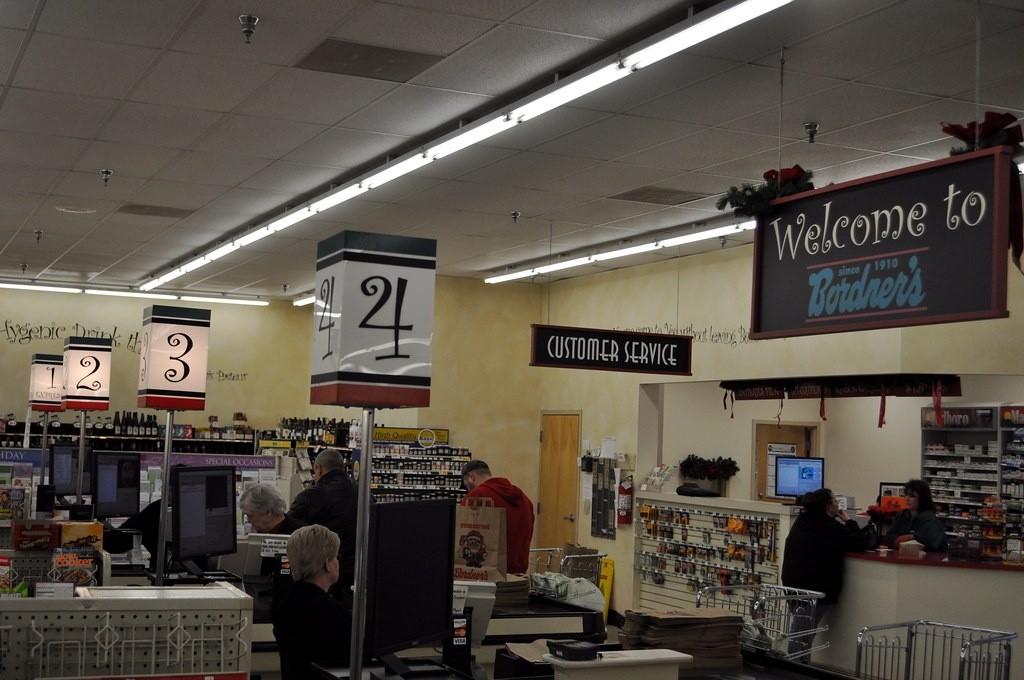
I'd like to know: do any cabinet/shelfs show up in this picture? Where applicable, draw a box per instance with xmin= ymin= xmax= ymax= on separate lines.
xmin=1001 ymin=407 xmax=1024 ymax=565
xmin=353 ymin=444 xmax=472 ymax=503
xmin=920 ymin=405 xmax=1000 ymax=550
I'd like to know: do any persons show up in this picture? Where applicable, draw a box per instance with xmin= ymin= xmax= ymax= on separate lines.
xmin=886 ymin=479 xmax=949 ymax=553
xmin=459 ymin=461 xmax=535 ymax=574
xmin=782 ymin=488 xmax=868 ymax=665
xmin=99 ymin=463 xmax=220 ymax=571
xmin=281 ymin=448 xmax=377 ymax=601
xmin=239 ymin=483 xmax=308 ymax=534
xmin=269 ymin=524 xmax=351 ymax=680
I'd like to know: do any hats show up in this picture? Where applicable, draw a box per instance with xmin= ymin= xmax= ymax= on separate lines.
xmin=459 ymin=460 xmax=490 ymax=490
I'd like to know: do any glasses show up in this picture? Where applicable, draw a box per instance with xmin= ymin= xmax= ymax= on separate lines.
xmin=311 ymin=467 xmax=317 ymax=478
xmin=245 ymin=513 xmax=259 ymax=523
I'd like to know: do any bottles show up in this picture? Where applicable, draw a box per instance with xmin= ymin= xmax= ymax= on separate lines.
xmin=0 ymin=436 xmax=57 ymax=448
xmin=126 ymin=412 xmax=132 ymax=437
xmin=132 ymin=412 xmax=139 ymax=437
xmin=6 ymin=413 xmax=18 ymax=433
xmin=276 ymin=417 xmax=362 ymax=449
xmin=85 ymin=415 xmax=93 ymax=435
xmin=372 ymin=492 xmax=467 ymax=506
xmin=74 ymin=416 xmax=81 ymax=434
xmin=146 ymin=414 xmax=158 ymax=437
xmin=95 ymin=416 xmax=104 ymax=436
xmin=158 ymin=412 xmax=254 ymax=440
xmin=113 ymin=411 xmax=121 ymax=435
xmin=38 ymin=415 xmax=48 ymax=434
xmin=342 ymin=452 xmax=354 ymax=479
xmin=120 ymin=412 xmax=128 ymax=436
xmin=371 ymin=459 xmax=468 ymax=470
xmin=373 ymin=440 xmax=469 ymax=456
xmin=50 ymin=415 xmax=61 ymax=434
xmin=371 ymin=471 xmax=463 ymax=486
xmin=140 ymin=413 xmax=145 ymax=437
xmin=105 ymin=417 xmax=114 ymax=436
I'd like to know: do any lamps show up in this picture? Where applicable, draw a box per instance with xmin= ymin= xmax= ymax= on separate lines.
xmin=0 ymin=0 xmax=791 ymax=305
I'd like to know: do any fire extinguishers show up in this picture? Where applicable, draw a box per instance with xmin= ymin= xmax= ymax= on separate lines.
xmin=618 ymin=475 xmax=632 ymax=524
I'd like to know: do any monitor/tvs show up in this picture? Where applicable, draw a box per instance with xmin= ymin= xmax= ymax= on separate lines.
xmin=48 ymin=446 xmax=140 ymax=532
xmin=775 ymin=457 xmax=825 ymax=505
xmin=167 ymin=465 xmax=241 ymax=582
xmin=878 ymin=482 xmax=906 ymax=509
xmin=362 ymin=498 xmax=455 ymax=680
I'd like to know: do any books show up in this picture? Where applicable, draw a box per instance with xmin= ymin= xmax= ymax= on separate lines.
xmin=491 ymin=573 xmax=529 ymax=607
xmin=452 ymin=579 xmax=498 ymax=649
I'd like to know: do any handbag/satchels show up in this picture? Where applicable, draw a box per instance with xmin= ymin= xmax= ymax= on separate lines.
xmin=530 ymin=571 xmax=606 ymax=612
